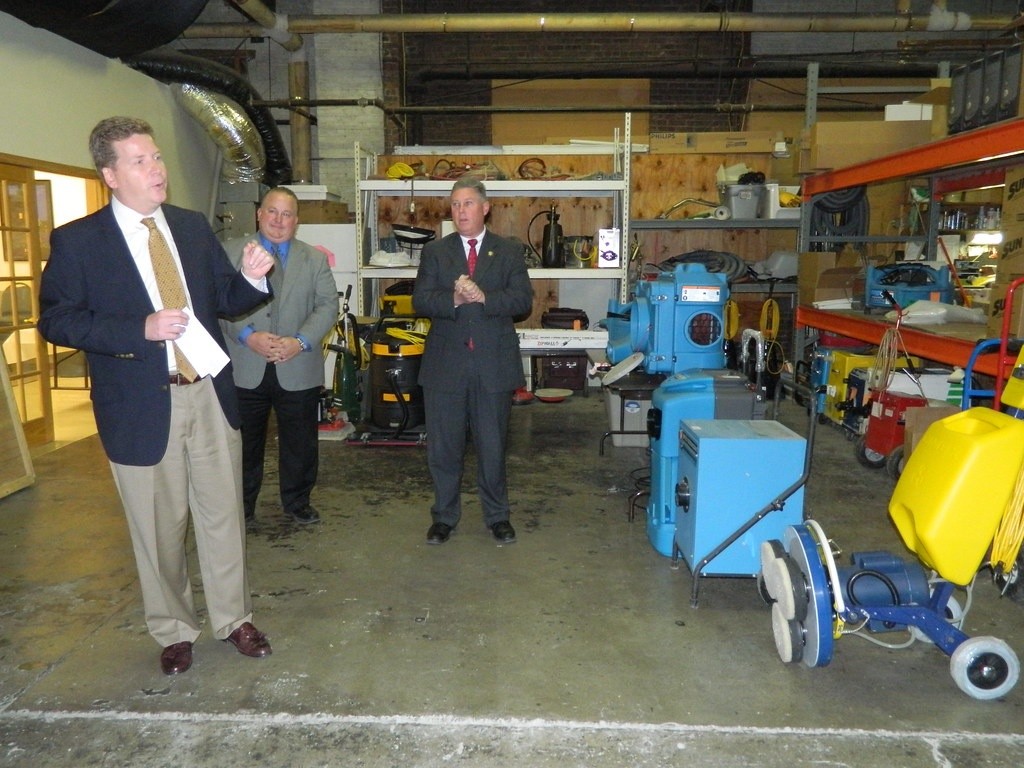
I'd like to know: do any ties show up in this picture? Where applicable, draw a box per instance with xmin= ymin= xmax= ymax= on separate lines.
xmin=140 ymin=217 xmax=200 ymax=383
xmin=466 ymin=238 xmax=478 ymax=349
xmin=268 ymin=245 xmax=284 ymax=343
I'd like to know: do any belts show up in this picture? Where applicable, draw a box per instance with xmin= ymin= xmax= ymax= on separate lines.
xmin=166 ymin=372 xmax=207 ymax=386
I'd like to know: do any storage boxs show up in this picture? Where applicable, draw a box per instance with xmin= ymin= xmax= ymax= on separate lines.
xmin=297 ymin=199 xmax=348 ymax=224
xmin=904 ymin=406 xmax=962 ymax=467
xmin=600 ymin=370 xmax=666 ymax=447
xmin=648 ymin=132 xmax=784 ymax=153
xmin=908 ymin=77 xmax=952 ymax=139
xmin=972 ymin=162 xmax=1024 ymax=340
xmin=884 ymin=104 xmax=933 ymax=120
xmin=811 ymin=120 xmax=934 ymax=170
xmin=278 ymin=185 xmax=342 ymax=201
xmin=593 ymin=228 xmax=620 ymax=268
xmin=718 ymin=183 xmax=762 ymax=218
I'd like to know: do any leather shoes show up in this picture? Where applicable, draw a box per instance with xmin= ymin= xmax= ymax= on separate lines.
xmin=218 ymin=620 xmax=274 ymax=660
xmin=161 ymin=639 xmax=194 ymax=675
xmin=491 ymin=519 xmax=514 ymax=540
xmin=243 ymin=503 xmax=257 ymax=520
xmin=286 ymin=503 xmax=324 ymax=523
xmin=427 ymin=518 xmax=456 ymax=544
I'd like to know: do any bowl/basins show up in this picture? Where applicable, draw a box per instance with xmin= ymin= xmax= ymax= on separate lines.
xmin=391 ymin=223 xmax=436 ymax=244
xmin=535 ymin=389 xmax=574 ymax=402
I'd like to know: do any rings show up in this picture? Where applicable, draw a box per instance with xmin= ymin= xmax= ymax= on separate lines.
xmin=264 ymin=250 xmax=269 ymax=255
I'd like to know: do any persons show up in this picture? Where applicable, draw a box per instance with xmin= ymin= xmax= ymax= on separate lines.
xmin=37 ymin=115 xmax=275 ymax=676
xmin=411 ymin=177 xmax=534 ymax=545
xmin=223 ymin=186 xmax=338 ymax=526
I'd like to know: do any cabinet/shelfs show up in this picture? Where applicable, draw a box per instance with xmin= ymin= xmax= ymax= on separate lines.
xmin=353 ymin=111 xmax=632 ymax=348
xmin=628 ymin=218 xmax=801 ymax=293
xmin=794 ymin=63 xmax=1024 ymax=384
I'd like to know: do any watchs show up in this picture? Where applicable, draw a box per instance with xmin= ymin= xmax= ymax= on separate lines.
xmin=297 ymin=337 xmax=307 ymax=351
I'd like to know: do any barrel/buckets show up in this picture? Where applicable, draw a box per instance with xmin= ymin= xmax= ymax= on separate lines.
xmin=563 ymin=235 xmax=593 ymax=269
